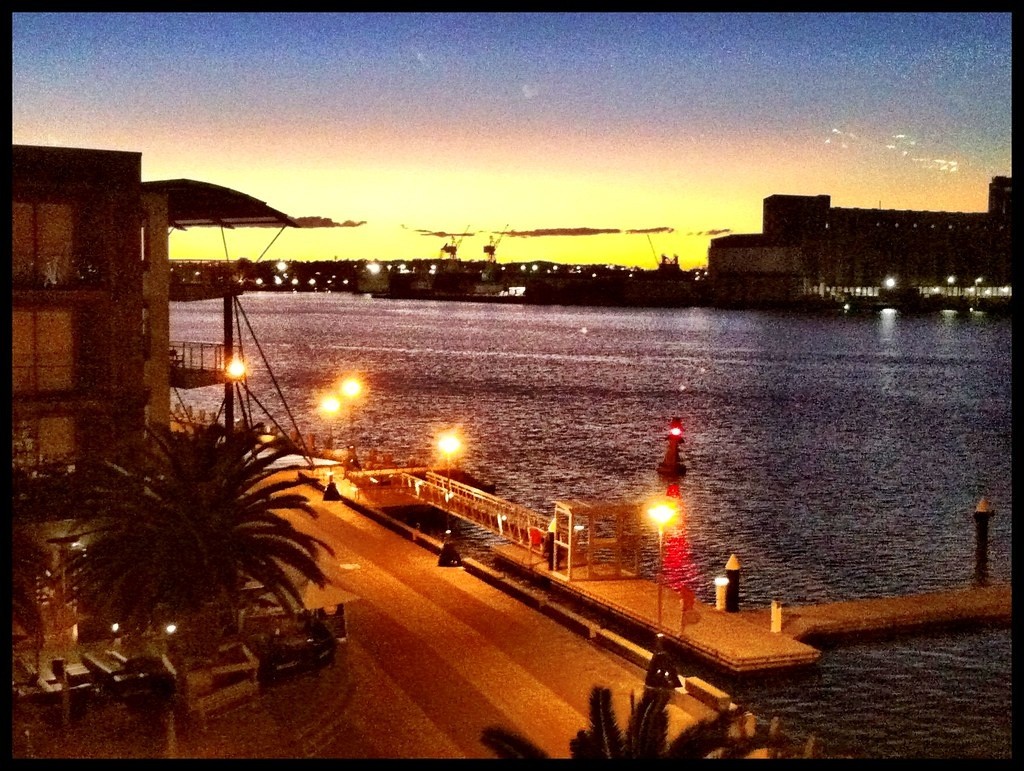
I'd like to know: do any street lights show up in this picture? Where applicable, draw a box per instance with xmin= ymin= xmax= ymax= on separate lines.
xmin=436 ymin=430 xmax=465 ymax=567
xmin=645 ymin=498 xmax=682 ymax=691
xmin=319 ymin=398 xmax=344 ymax=502
xmin=340 ymin=379 xmax=364 ymax=475
